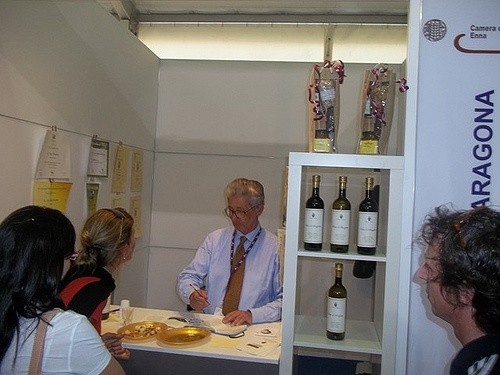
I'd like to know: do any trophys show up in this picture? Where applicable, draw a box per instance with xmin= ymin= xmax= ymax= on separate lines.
xmin=360 ymin=82 xmax=387 ymax=155
xmin=312 ymin=78 xmax=336 ymax=152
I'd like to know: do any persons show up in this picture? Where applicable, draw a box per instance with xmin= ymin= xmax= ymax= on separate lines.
xmin=176 ymin=178 xmax=283 ymax=327
xmin=0 ymin=205 xmax=125 ymax=375
xmin=416 ymin=208 xmax=500 ymax=375
xmin=56 ymin=207 xmax=136 ymax=354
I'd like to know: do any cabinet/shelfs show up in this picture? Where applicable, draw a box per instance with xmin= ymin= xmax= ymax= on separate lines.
xmin=279 ymin=152 xmax=405 ymax=375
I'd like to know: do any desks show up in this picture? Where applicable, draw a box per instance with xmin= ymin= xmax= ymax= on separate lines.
xmin=100 ymin=304 xmax=282 ymax=375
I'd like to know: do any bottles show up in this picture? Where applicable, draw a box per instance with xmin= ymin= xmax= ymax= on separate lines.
xmin=357 ymin=177 xmax=379 ymax=256
xmin=326 ymin=263 xmax=348 ymax=341
xmin=330 ymin=176 xmax=351 ymax=253
xmin=303 ymin=175 xmax=324 ymax=251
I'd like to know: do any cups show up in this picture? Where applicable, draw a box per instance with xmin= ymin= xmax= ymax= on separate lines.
xmin=119 ymin=299 xmax=130 ymax=316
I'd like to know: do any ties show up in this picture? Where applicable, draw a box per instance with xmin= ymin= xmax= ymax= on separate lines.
xmin=222 ymin=235 xmax=248 ymax=315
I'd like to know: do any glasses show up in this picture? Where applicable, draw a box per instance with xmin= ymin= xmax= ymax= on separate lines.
xmin=100 ymin=207 xmax=126 ymax=222
xmin=224 ymin=203 xmax=260 ymax=218
xmin=64 ymin=250 xmax=79 ymax=261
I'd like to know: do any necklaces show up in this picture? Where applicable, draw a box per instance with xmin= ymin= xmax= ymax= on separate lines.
xmin=230 ymin=228 xmax=262 ymax=273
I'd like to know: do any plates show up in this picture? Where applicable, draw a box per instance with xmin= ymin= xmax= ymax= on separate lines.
xmin=117 ymin=321 xmax=167 ymax=342
xmin=156 ymin=326 xmax=211 ymax=347
